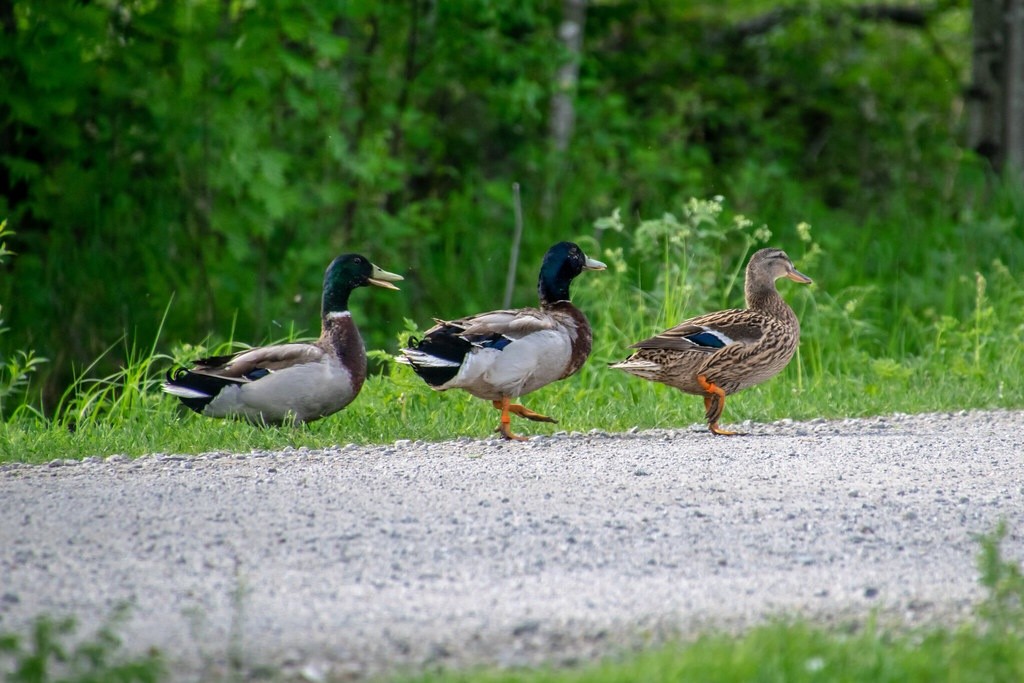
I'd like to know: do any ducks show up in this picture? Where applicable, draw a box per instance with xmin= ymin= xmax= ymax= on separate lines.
xmin=160 ymin=253 xmax=406 ymax=432
xmin=612 ymin=246 xmax=812 ymax=436
xmin=394 ymin=240 xmax=608 ymax=441
